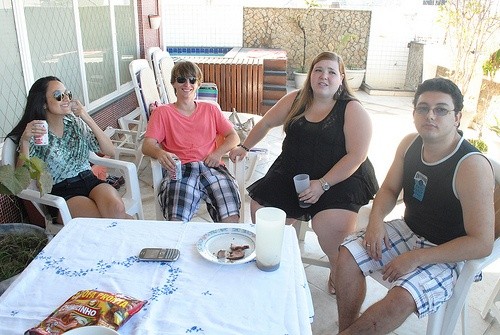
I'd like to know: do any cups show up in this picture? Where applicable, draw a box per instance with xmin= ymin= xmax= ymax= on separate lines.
xmin=294 ymin=174 xmax=313 ymax=208
xmin=253 ymin=207 xmax=286 ymax=272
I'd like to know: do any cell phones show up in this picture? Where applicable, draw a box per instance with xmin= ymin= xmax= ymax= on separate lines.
xmin=138 ymin=248 xmax=180 ymax=262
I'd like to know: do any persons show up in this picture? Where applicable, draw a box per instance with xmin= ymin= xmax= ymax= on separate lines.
xmin=229 ymin=52 xmax=379 ymax=294
xmin=142 ymin=60 xmax=242 ymax=223
xmin=337 ymin=78 xmax=496 ymax=335
xmin=4 ymin=76 xmax=133 ymax=220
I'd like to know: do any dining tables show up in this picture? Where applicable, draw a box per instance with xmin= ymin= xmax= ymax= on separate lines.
xmin=0 ymin=217 xmax=314 ymax=335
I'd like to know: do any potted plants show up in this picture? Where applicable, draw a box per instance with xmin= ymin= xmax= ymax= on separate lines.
xmin=296 ymin=3 xmax=308 ymax=88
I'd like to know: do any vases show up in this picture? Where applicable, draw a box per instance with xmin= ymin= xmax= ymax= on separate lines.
xmin=346 ymin=68 xmax=365 ymax=90
xmin=0 ymin=224 xmax=52 ymax=294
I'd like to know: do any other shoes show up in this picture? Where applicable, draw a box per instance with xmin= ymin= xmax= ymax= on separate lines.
xmin=105 ymin=175 xmax=125 ymax=191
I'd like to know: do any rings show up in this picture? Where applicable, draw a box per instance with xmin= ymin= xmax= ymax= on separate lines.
xmin=163 ymin=162 xmax=165 ymax=165
xmin=366 ymin=243 xmax=371 ymax=246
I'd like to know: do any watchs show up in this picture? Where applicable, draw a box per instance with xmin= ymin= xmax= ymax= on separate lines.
xmin=319 ymin=178 xmax=330 ymax=192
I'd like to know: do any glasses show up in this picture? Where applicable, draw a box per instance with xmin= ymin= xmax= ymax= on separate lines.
xmin=46 ymin=89 xmax=72 ymax=101
xmin=174 ymin=76 xmax=200 ymax=85
xmin=414 ymin=107 xmax=456 ymax=117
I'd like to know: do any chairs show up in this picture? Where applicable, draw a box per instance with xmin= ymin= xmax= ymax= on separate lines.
xmin=424 ymin=158 xmax=500 ymax=335
xmin=2 ymin=47 xmax=374 ymax=266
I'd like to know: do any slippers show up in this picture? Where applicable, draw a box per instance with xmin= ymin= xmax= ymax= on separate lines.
xmin=328 ymin=272 xmax=336 ymax=295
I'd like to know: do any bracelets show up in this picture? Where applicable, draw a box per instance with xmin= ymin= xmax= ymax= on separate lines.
xmin=241 ymin=145 xmax=249 ymax=152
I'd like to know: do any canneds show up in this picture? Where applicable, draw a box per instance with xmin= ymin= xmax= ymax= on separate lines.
xmin=34 ymin=120 xmax=48 ymax=145
xmin=169 ymin=158 xmax=181 ymax=180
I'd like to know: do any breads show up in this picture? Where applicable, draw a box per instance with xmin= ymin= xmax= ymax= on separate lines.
xmin=219 ymin=245 xmax=249 ymax=260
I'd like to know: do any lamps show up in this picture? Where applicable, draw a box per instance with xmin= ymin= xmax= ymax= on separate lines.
xmin=149 ymin=15 xmax=161 ymax=29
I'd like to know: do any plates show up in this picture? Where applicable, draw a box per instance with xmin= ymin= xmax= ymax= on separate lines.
xmin=196 ymin=229 xmax=255 ymax=265
xmin=59 ymin=325 xmax=121 ymax=335
